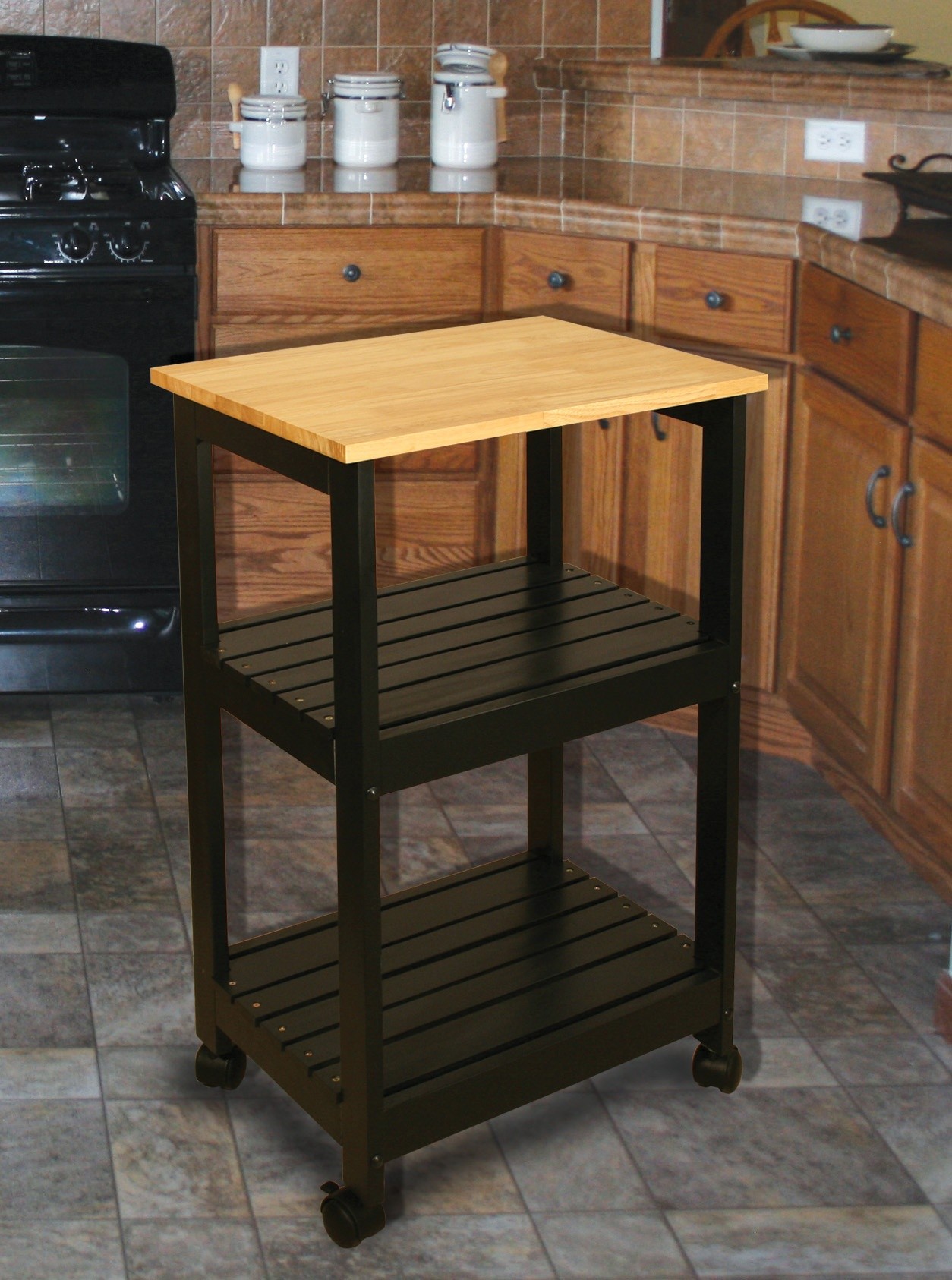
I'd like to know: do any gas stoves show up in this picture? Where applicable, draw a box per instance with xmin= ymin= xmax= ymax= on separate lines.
xmin=0 ymin=34 xmax=197 ymax=266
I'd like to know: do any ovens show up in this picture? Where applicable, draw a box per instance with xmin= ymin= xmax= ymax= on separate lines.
xmin=0 ymin=267 xmax=190 ymax=695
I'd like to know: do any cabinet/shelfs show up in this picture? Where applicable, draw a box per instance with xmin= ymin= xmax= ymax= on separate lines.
xmin=189 ymin=220 xmax=952 ymax=1045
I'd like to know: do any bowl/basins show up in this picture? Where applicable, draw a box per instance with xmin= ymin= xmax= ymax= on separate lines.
xmin=789 ymin=23 xmax=895 ymax=53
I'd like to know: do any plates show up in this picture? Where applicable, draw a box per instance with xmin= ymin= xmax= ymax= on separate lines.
xmin=765 ymin=41 xmax=918 ymax=64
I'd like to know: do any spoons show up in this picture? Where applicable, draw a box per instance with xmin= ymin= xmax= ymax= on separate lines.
xmin=227 ymin=82 xmax=243 ymax=149
xmin=488 ymin=52 xmax=508 ymax=143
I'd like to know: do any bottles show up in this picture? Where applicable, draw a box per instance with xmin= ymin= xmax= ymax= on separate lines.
xmin=429 ymin=41 xmax=509 ymax=168
xmin=230 ymin=93 xmax=308 ymax=171
xmin=319 ymin=73 xmax=404 ymax=168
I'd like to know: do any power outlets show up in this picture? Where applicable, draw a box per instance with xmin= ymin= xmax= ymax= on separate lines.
xmin=798 ymin=193 xmax=864 ymax=243
xmin=801 ymin=116 xmax=868 ymax=166
